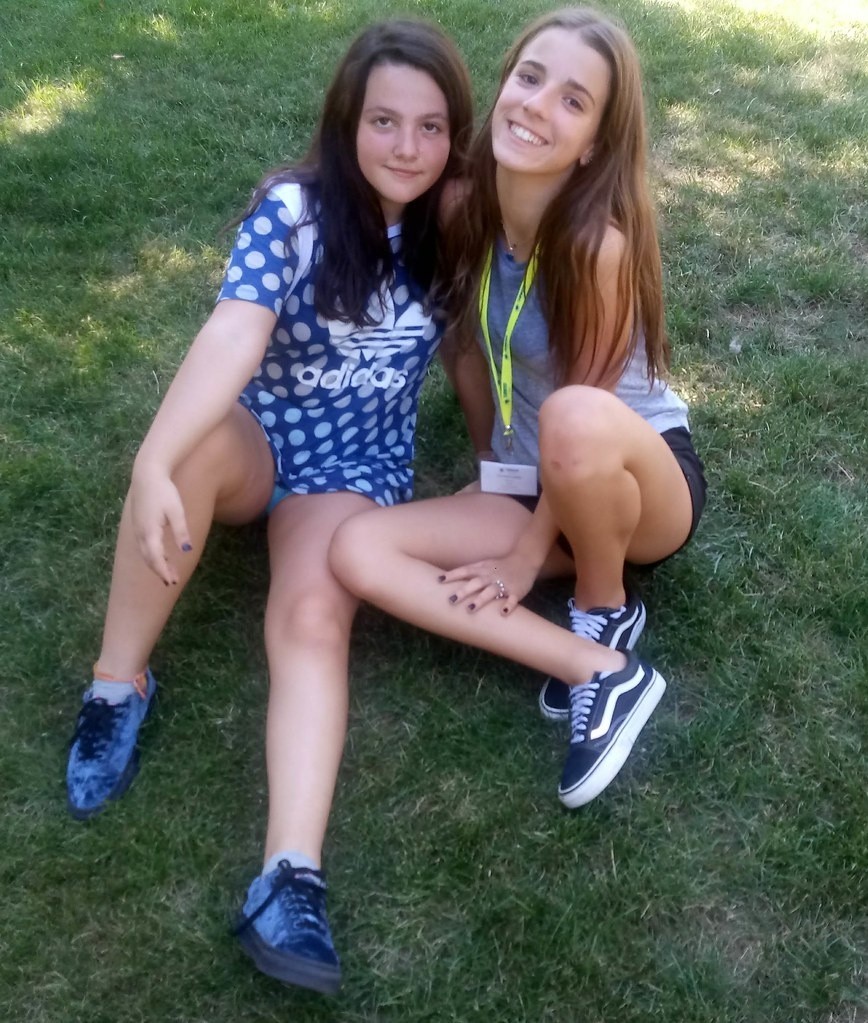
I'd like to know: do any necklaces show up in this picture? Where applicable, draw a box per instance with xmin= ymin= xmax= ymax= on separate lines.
xmin=504 ymin=227 xmax=534 ymax=251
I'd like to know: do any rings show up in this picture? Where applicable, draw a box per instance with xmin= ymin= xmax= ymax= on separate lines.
xmin=493 ymin=580 xmax=505 ymax=600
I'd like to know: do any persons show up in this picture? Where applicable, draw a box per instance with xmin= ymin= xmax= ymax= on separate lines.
xmin=327 ymin=8 xmax=705 ymax=806
xmin=67 ymin=22 xmax=480 ymax=987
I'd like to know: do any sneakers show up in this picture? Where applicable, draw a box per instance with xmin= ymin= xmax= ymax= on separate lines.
xmin=61 ymin=667 xmax=161 ymax=821
xmin=557 ymin=649 xmax=667 ymax=810
xmin=228 ymin=859 xmax=342 ymax=996
xmin=539 ymin=581 xmax=647 ymax=719
